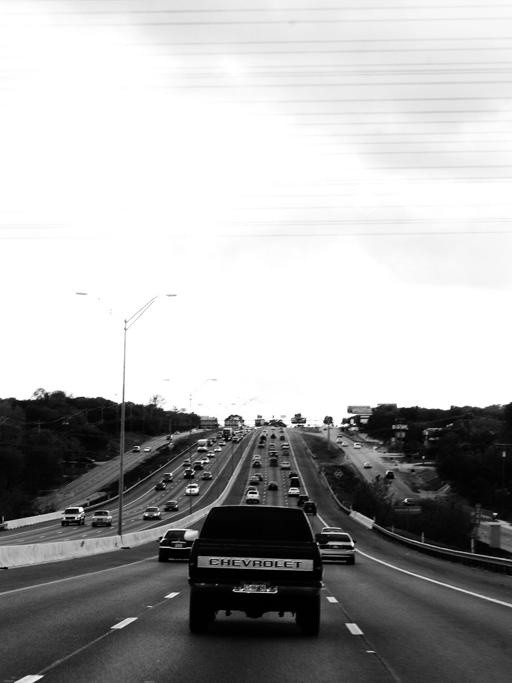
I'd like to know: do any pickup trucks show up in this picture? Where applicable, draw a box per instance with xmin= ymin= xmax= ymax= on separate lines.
xmin=186 ymin=503 xmax=325 ymax=636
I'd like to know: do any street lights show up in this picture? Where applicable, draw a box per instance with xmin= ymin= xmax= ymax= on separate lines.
xmin=117 ymin=292 xmax=178 ymax=536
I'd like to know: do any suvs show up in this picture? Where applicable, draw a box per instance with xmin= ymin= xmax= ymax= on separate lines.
xmin=60 ymin=506 xmax=85 ymax=526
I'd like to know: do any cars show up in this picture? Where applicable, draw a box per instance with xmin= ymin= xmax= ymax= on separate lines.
xmin=164 ymin=500 xmax=179 ymax=512
xmin=131 ymin=445 xmax=152 ymax=453
xmin=155 ymin=427 xmax=244 ymax=496
xmin=244 ymin=426 xmax=318 ymax=516
xmin=335 ymin=433 xmax=395 ymax=480
xmin=404 ymin=498 xmax=414 ymax=503
xmin=91 ymin=509 xmax=112 ymax=527
xmin=158 ymin=527 xmax=199 ymax=562
xmin=316 ymin=526 xmax=357 ymax=566
xmin=143 ymin=506 xmax=161 ymax=520
